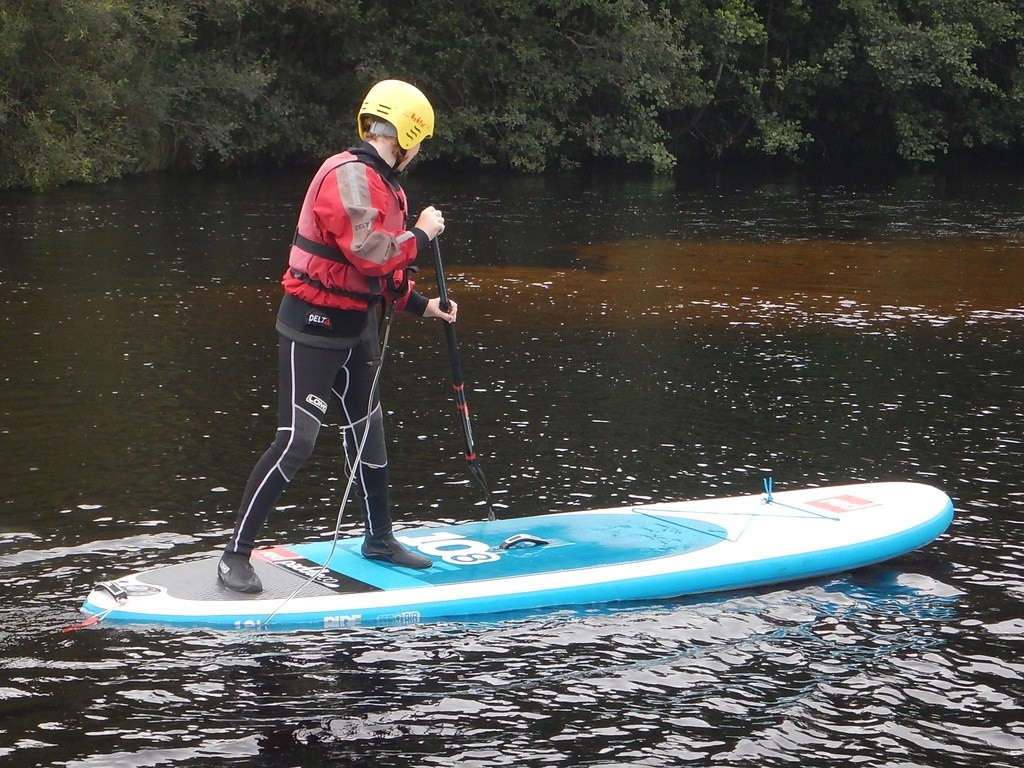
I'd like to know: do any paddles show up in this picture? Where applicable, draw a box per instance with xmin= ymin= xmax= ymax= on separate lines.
xmin=432 ymin=235 xmax=495 ymax=508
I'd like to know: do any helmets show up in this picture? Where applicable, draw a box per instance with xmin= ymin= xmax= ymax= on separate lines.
xmin=356 ymin=79 xmax=435 ymax=150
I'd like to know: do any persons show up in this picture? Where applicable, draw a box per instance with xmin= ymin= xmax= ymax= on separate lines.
xmin=218 ymin=79 xmax=458 ymax=594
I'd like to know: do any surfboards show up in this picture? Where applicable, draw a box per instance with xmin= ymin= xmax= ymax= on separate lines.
xmin=82 ymin=479 xmax=955 ymax=634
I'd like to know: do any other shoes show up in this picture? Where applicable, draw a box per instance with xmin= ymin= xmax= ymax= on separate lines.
xmin=360 ymin=530 xmax=432 ymax=568
xmin=217 ymin=553 xmax=262 ymax=593
